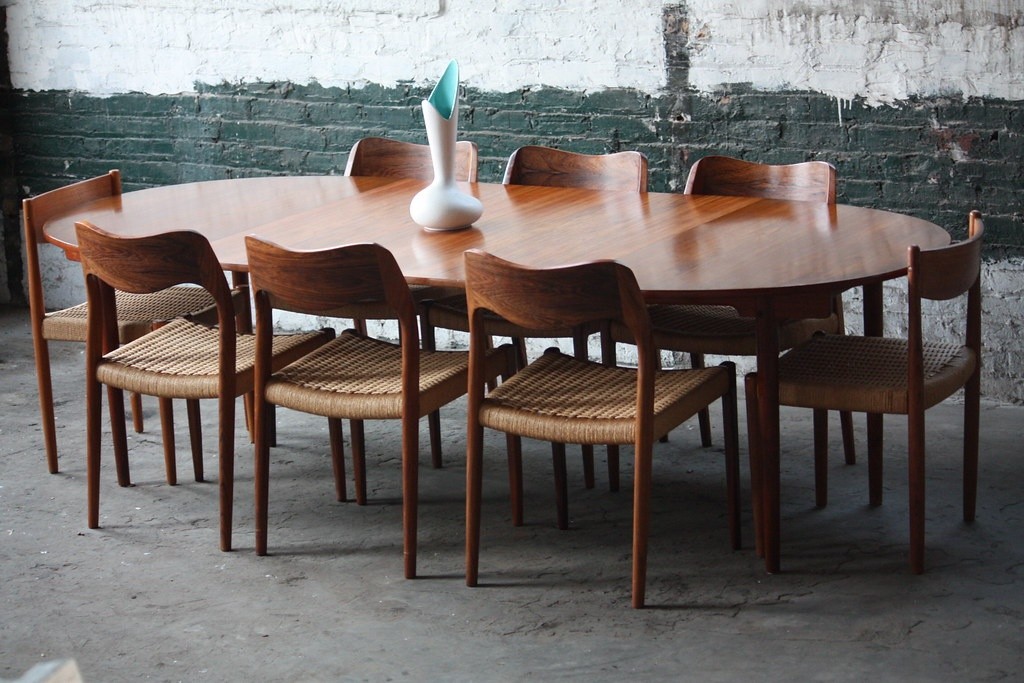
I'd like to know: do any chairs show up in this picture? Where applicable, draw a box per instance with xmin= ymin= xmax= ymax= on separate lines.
xmin=22 ymin=169 xmax=255 ymax=493
xmin=73 ymin=219 xmax=349 ymax=554
xmin=744 ymin=209 xmax=985 ymax=575
xmin=242 ymin=136 xmax=854 ymax=611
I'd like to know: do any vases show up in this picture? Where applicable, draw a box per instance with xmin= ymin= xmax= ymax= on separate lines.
xmin=409 ymin=58 xmax=483 ymax=232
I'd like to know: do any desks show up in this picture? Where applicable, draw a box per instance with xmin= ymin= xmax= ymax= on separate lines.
xmin=44 ymin=176 xmax=952 ymax=575
